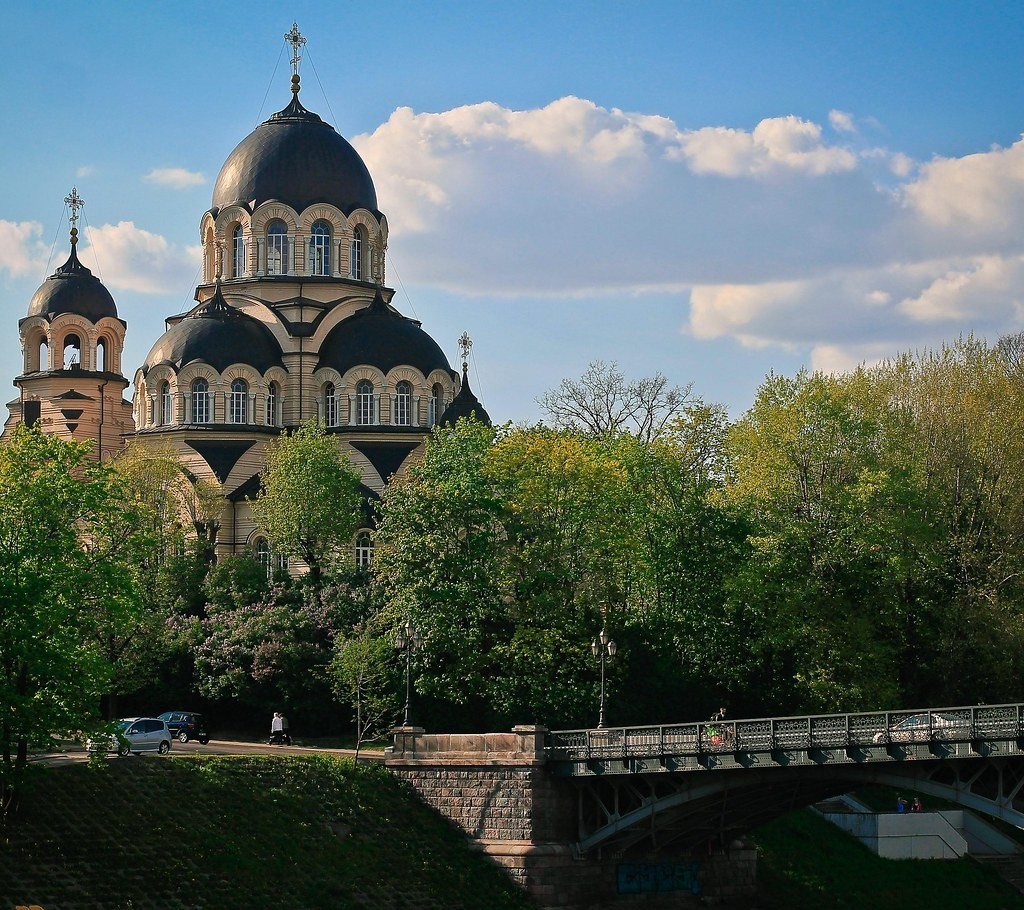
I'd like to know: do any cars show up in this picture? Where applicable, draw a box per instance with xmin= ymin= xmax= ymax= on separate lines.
xmin=154 ymin=711 xmax=210 ymax=745
xmin=872 ymin=714 xmax=974 ymax=743
xmin=87 ymin=717 xmax=172 ymax=757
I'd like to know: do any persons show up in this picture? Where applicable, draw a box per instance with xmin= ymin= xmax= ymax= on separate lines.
xmin=710 ymin=707 xmax=733 ymax=735
xmin=271 ymin=712 xmax=294 ymax=746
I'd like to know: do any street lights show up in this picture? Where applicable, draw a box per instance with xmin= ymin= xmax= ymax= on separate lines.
xmin=592 ymin=625 xmax=617 ymax=744
xmin=394 ymin=617 xmax=425 ymax=750
